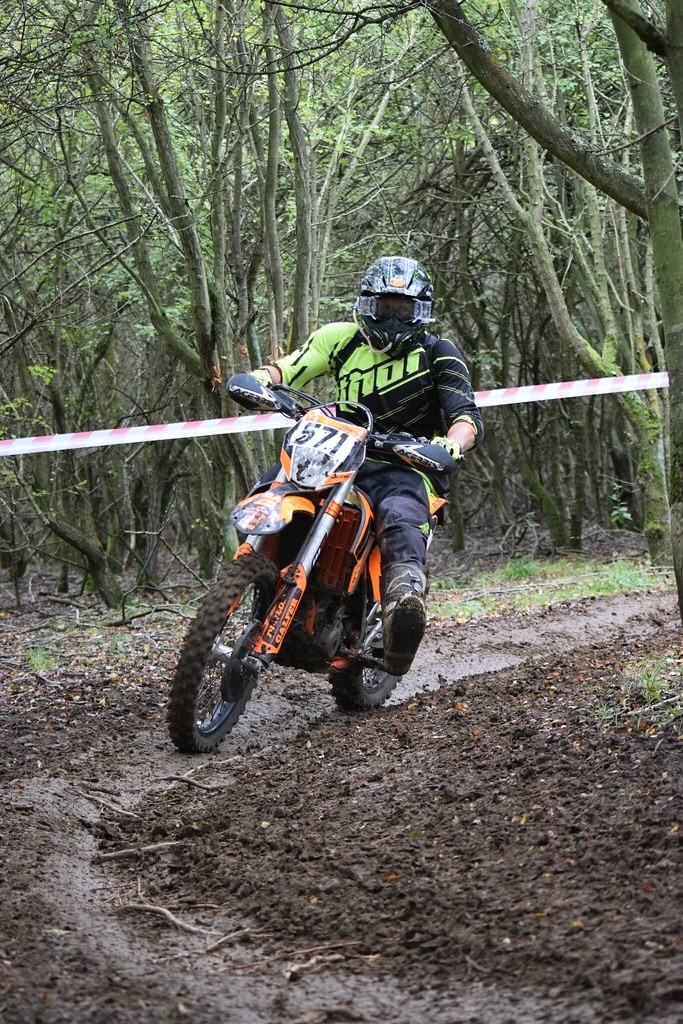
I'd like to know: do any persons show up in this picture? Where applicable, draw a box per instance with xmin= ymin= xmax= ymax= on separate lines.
xmin=243 ymin=255 xmax=485 ymax=678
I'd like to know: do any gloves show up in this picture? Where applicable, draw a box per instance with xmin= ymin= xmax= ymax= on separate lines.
xmin=250 ymin=370 xmax=272 ymax=387
xmin=431 ymin=437 xmax=462 ymax=463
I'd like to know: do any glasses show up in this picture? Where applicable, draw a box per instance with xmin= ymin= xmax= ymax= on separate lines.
xmin=369 ymin=294 xmax=421 ymax=323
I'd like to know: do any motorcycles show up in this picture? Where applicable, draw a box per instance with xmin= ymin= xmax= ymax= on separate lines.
xmin=164 ymin=373 xmax=465 ymax=754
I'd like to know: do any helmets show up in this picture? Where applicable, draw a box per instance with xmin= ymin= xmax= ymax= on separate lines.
xmin=361 ymin=255 xmax=433 ymax=358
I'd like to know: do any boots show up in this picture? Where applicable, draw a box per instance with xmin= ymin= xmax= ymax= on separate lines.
xmin=380 ymin=563 xmax=426 ymax=675
